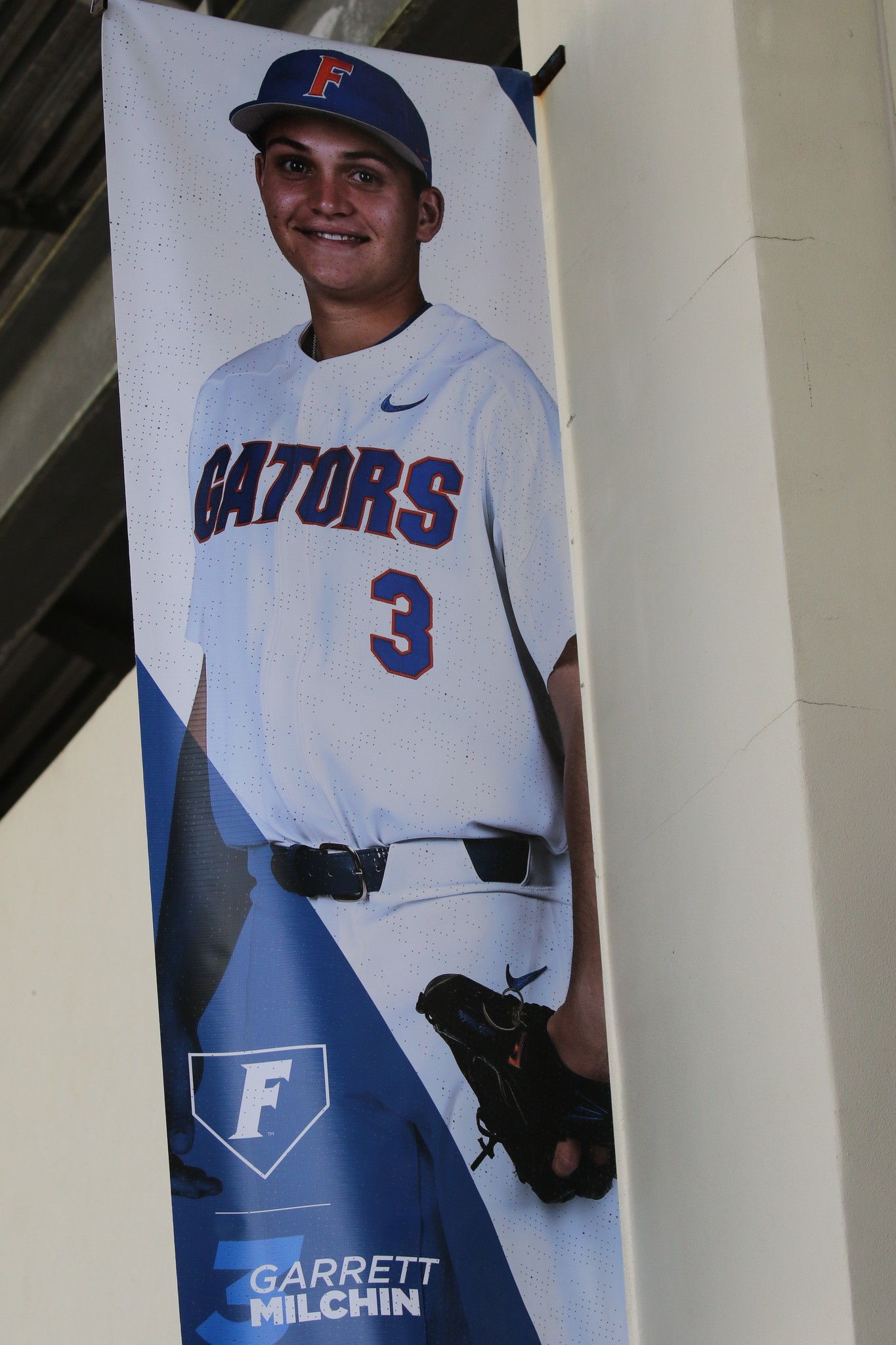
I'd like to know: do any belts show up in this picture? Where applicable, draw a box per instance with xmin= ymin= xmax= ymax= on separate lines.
xmin=270 ymin=829 xmax=529 ymax=902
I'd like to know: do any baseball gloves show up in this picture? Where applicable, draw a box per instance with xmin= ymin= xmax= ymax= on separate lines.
xmin=416 ymin=972 xmax=617 ymax=1206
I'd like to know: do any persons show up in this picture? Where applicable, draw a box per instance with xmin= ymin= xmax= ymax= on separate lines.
xmin=154 ymin=47 xmax=634 ymax=1345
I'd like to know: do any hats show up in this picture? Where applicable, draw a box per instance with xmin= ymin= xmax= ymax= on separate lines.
xmin=229 ymin=49 xmax=432 ymax=188
